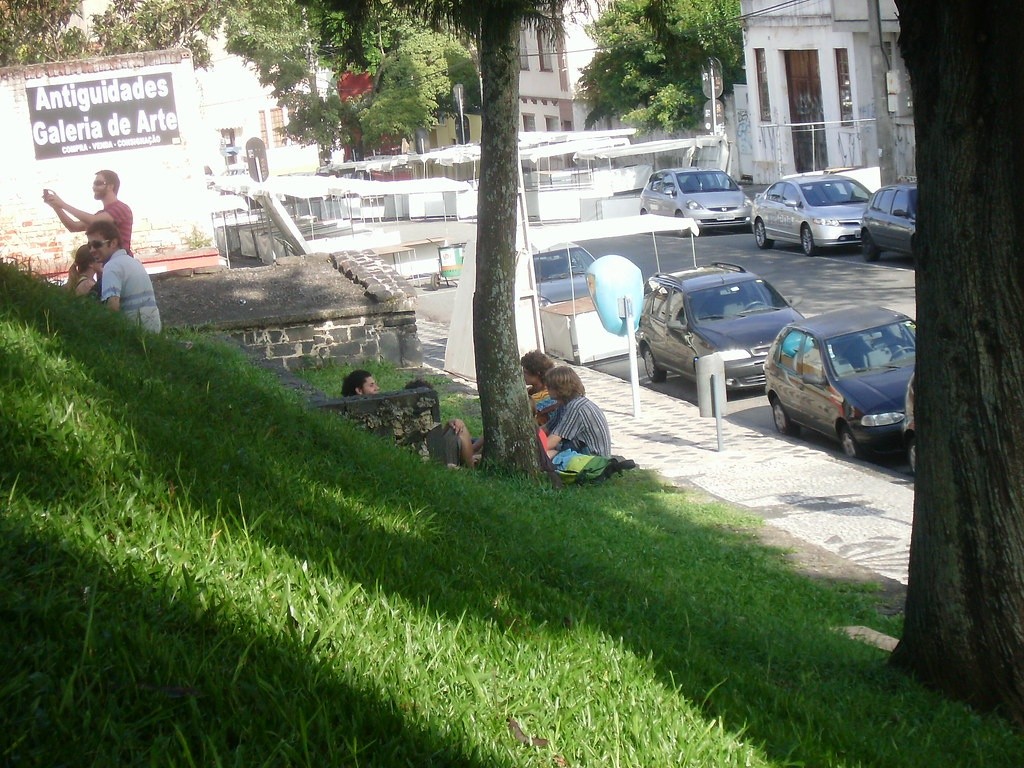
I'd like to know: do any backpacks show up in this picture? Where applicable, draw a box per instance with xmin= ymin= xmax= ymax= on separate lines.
xmin=550 ymin=446 xmax=636 ymax=488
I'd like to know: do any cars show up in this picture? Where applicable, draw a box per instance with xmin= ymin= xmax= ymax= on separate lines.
xmin=639 ymin=167 xmax=753 ymax=236
xmin=750 ymin=174 xmax=871 ymax=256
xmin=763 ymin=306 xmax=917 ymax=472
xmin=860 ymin=182 xmax=918 ymax=262
xmin=533 ymin=241 xmax=597 ymax=308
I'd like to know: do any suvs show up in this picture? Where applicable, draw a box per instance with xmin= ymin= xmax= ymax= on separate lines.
xmin=634 ymin=260 xmax=806 ymax=395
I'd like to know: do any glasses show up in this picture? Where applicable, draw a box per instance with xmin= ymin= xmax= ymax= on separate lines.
xmin=93 ymin=180 xmax=107 ymax=186
xmin=87 ymin=240 xmax=110 ymax=250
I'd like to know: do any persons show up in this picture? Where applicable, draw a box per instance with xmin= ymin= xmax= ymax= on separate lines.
xmin=472 ymin=351 xmax=555 ymax=454
xmin=85 ymin=219 xmax=162 ymax=334
xmin=42 ymin=170 xmax=133 ymax=281
xmin=550 ymin=366 xmax=611 ymax=441
xmin=405 ymin=379 xmax=474 ymax=469
xmin=341 ymin=370 xmax=380 ymax=397
xmin=63 ymin=244 xmax=103 ymax=296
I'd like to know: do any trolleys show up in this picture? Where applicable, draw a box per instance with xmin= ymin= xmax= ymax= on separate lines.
xmin=425 ymin=237 xmax=466 ymax=290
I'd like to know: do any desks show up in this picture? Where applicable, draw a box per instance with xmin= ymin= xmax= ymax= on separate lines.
xmin=393 ymin=237 xmax=448 ymax=272
xmin=372 ymin=245 xmax=422 ymax=288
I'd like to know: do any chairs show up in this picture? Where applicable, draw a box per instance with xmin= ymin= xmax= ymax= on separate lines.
xmin=859 ymin=332 xmax=895 ymax=368
xmin=714 ymin=286 xmax=745 ymax=318
xmin=831 ymin=353 xmax=856 ymax=377
